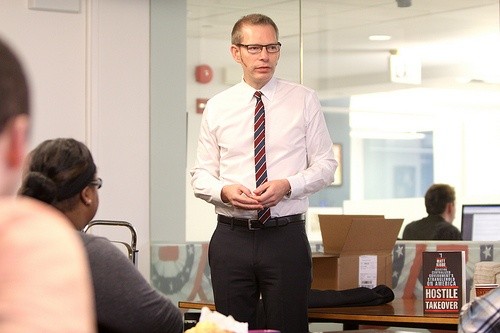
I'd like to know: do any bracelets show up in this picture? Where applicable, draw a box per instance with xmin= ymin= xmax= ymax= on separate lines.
xmin=288 ymin=187 xmax=291 ymax=195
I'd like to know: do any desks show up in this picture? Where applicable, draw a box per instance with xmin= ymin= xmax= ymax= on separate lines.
xmin=179 ymin=300 xmax=459 ymax=324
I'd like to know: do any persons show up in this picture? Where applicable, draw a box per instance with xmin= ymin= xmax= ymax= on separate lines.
xmin=402 ymin=184 xmax=461 ymax=240
xmin=190 ymin=14 xmax=338 ymax=333
xmin=0 ymin=40 xmax=97 ymax=333
xmin=16 ymin=138 xmax=183 ymax=333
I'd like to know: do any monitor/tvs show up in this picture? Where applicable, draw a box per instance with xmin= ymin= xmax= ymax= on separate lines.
xmin=461 ymin=204 xmax=500 ymax=241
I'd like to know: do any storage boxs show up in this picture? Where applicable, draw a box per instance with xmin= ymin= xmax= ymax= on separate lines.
xmin=313 ymin=214 xmax=405 ymax=290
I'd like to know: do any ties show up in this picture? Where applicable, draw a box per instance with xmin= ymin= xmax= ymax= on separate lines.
xmin=253 ymin=91 xmax=271 ymax=225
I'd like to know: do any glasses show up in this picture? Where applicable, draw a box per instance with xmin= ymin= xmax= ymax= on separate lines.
xmin=90 ymin=178 xmax=103 ymax=190
xmin=237 ymin=42 xmax=282 ymax=54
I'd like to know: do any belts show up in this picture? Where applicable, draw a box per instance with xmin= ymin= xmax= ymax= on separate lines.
xmin=216 ymin=214 xmax=306 ymax=231
